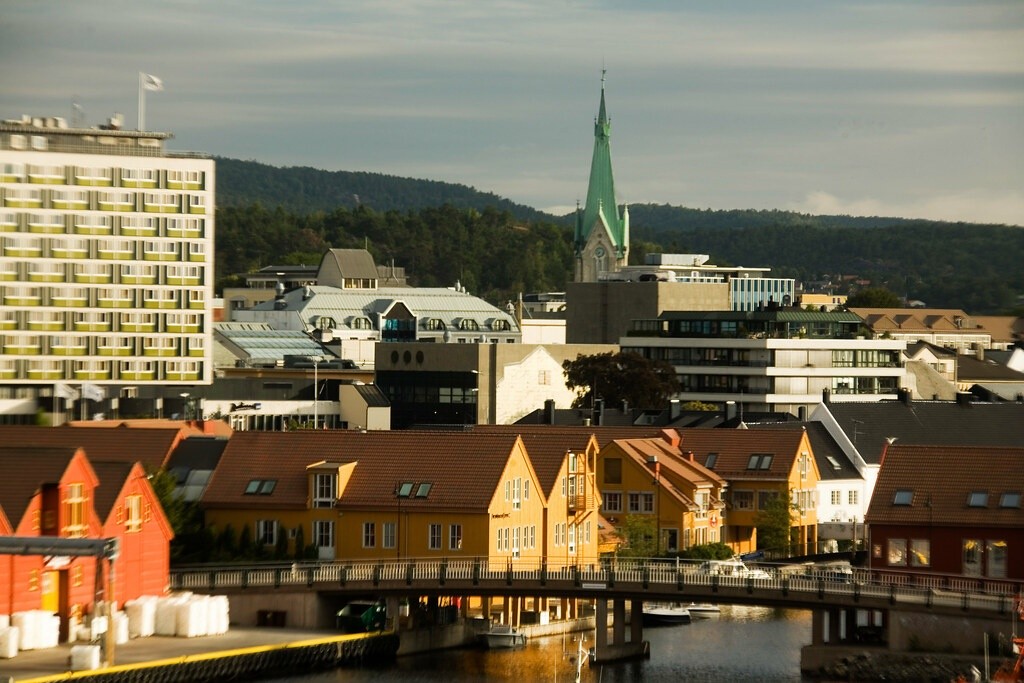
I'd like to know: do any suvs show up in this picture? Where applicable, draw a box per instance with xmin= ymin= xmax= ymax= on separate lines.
xmin=686 ymin=560 xmax=749 ymax=581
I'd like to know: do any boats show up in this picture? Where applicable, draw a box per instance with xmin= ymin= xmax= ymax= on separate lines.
xmin=643 ymin=607 xmax=697 ymax=624
xmin=686 ymin=602 xmax=720 ymax=618
xmin=476 ymin=625 xmax=527 ymax=648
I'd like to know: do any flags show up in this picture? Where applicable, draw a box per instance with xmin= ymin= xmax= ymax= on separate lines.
xmin=142 ymin=73 xmax=164 ymax=91
